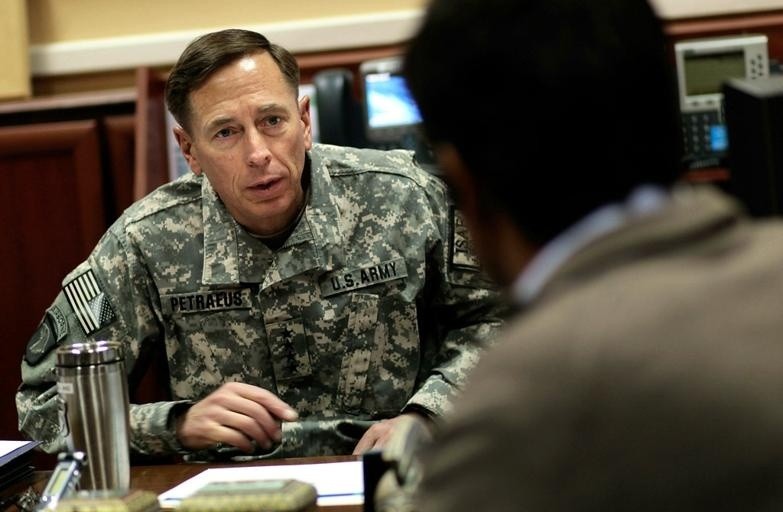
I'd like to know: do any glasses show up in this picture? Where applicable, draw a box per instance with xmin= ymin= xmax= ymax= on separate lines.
xmin=401 ymin=116 xmax=445 ymax=192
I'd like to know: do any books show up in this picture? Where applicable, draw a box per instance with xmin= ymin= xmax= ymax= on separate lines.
xmin=0 ymin=438 xmax=43 ymax=468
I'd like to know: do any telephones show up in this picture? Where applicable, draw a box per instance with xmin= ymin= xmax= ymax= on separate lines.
xmin=674 ymin=34 xmax=771 ymax=171
xmin=316 ymin=56 xmax=447 ymax=178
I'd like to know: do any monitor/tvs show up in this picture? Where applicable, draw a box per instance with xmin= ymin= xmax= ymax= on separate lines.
xmin=359 ymin=57 xmax=424 ymax=144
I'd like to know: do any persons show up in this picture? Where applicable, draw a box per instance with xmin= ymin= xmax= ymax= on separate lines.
xmin=372 ymin=1 xmax=782 ymax=511
xmin=12 ymin=29 xmax=512 ymax=462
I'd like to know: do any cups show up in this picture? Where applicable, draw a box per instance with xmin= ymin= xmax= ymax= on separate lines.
xmin=54 ymin=340 xmax=132 ymax=500
